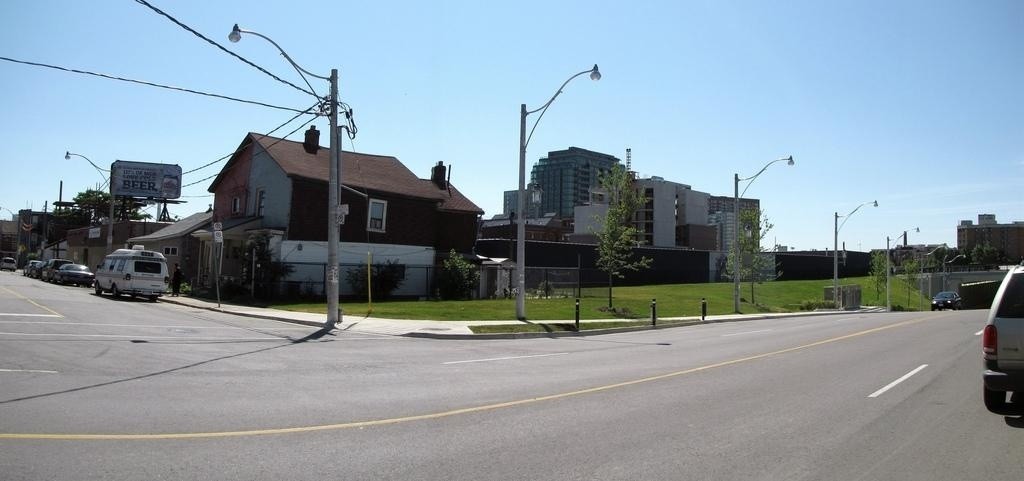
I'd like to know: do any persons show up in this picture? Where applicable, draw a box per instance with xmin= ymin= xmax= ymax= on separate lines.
xmin=172 ymin=263 xmax=184 ymax=297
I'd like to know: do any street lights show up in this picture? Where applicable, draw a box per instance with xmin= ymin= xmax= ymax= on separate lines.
xmin=228 ymin=24 xmax=340 ymax=325
xmin=65 ymin=148 xmax=118 ymax=255
xmin=833 ymin=198 xmax=878 ymax=308
xmin=942 ymin=253 xmax=967 ymax=291
xmin=920 ymin=242 xmax=948 ymax=304
xmin=516 ymin=62 xmax=602 ymax=320
xmin=733 ymin=154 xmax=794 ymax=315
xmin=885 ymin=226 xmax=921 ymax=312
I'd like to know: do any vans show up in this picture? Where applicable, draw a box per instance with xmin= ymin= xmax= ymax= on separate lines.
xmin=94 ymin=244 xmax=171 ymax=301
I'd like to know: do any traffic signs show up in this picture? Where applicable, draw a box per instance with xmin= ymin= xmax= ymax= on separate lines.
xmin=0 ymin=202 xmax=22 ymax=264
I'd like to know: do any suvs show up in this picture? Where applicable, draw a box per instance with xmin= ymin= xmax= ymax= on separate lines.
xmin=982 ymin=257 xmax=1024 ymax=412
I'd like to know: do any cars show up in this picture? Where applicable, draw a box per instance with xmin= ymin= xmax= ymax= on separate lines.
xmin=22 ymin=257 xmax=95 ymax=288
xmin=931 ymin=289 xmax=962 ymax=311
xmin=0 ymin=256 xmax=18 ymax=272
